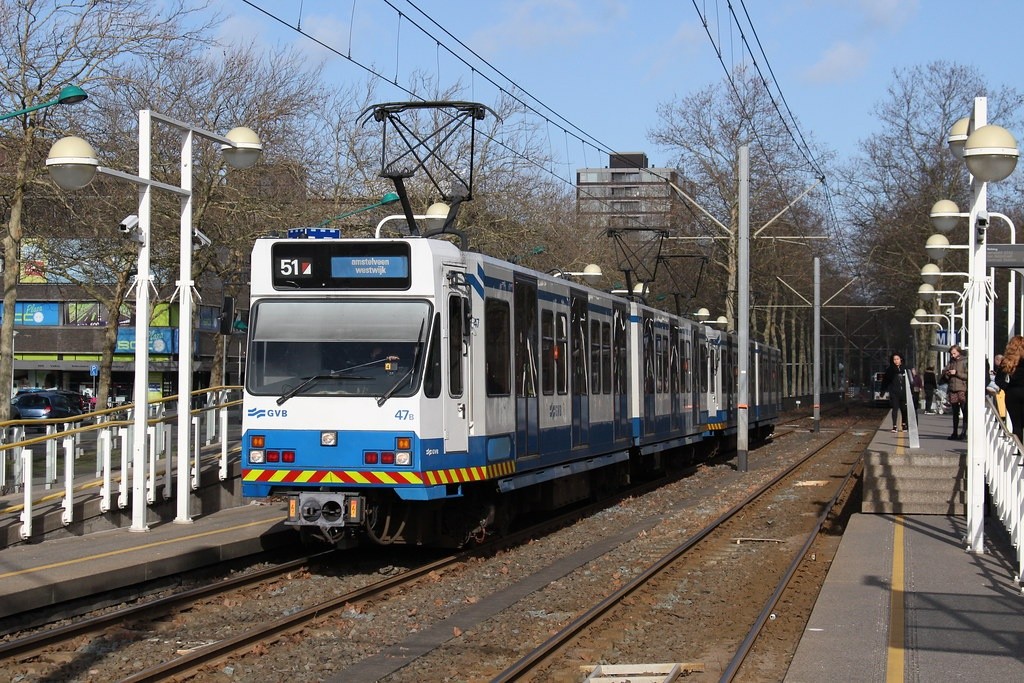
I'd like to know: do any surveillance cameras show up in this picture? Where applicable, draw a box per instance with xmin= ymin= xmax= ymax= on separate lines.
xmin=946 ymin=309 xmax=951 ymax=315
xmin=976 ymin=211 xmax=989 ymax=229
xmin=193 ymin=228 xmax=211 ymax=247
xmin=118 ymin=215 xmax=139 ymax=233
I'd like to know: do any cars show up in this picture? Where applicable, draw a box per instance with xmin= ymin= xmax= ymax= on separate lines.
xmin=9 ymin=388 xmax=127 ymax=430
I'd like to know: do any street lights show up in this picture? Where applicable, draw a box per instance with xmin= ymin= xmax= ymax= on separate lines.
xmin=907 ymin=94 xmax=1019 ymax=554
xmin=46 ymin=110 xmax=264 ymax=532
xmin=10 ymin=329 xmax=19 ymax=399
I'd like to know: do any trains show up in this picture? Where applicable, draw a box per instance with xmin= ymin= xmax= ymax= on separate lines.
xmin=871 ymin=372 xmax=891 ymax=402
xmin=213 ymin=101 xmax=785 ymax=548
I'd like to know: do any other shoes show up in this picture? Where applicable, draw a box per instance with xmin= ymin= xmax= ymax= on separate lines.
xmin=902 ymin=424 xmax=908 ymax=433
xmin=924 ymin=410 xmax=936 ymax=415
xmin=891 ymin=426 xmax=898 ymax=433
xmin=947 ymin=433 xmax=957 ymax=440
xmin=957 ymin=433 xmax=968 ymax=440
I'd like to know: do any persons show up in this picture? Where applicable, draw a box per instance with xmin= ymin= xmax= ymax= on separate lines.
xmin=985 ymin=354 xmax=991 ymax=388
xmin=995 ymin=335 xmax=1024 ymax=466
xmin=991 ymin=354 xmax=1006 ymax=430
xmin=880 ymin=352 xmax=922 ymax=432
xmin=940 ymin=344 xmax=968 ymax=440
xmin=924 ymin=365 xmax=938 ymax=414
xmin=350 ymin=342 xmax=401 ymax=368
